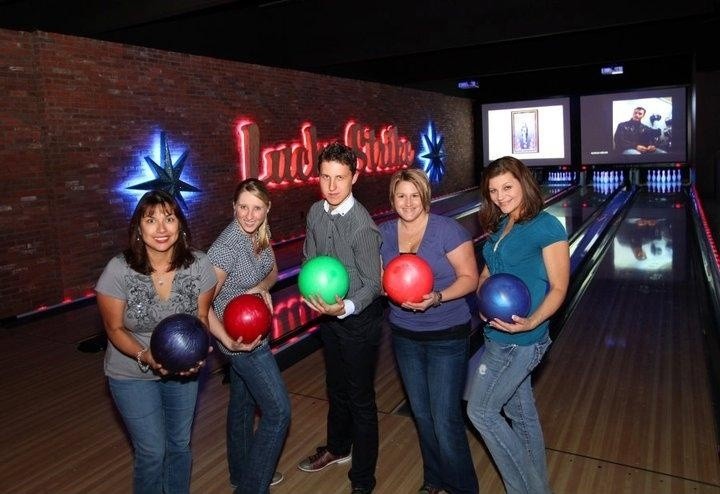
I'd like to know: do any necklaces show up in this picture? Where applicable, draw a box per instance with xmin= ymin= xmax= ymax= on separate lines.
xmin=148 ymin=255 xmax=170 ymax=286
xmin=400 ymin=212 xmax=426 ymax=245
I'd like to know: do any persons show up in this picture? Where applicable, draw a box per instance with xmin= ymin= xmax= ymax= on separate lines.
xmin=614 ymin=106 xmax=671 ymax=156
xmin=206 ymin=177 xmax=291 ymax=494
xmin=96 ymin=190 xmax=216 ymax=494
xmin=616 ymin=213 xmax=672 ymax=265
xmin=292 ymin=143 xmax=386 ymax=493
xmin=518 ymin=124 xmax=531 ymax=148
xmin=460 ymin=156 xmax=570 ymax=494
xmin=374 ymin=165 xmax=482 ymax=494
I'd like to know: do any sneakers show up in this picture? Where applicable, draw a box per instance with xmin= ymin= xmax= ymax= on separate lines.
xmin=417 ymin=480 xmax=444 ymax=494
xmin=349 ymin=476 xmax=376 ymax=494
xmin=297 ymin=447 xmax=352 ymax=472
xmin=230 ymin=471 xmax=284 ymax=488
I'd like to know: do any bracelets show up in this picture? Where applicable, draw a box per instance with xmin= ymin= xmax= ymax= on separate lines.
xmin=433 ymin=291 xmax=442 ymax=308
xmin=136 ymin=347 xmax=151 ymax=373
